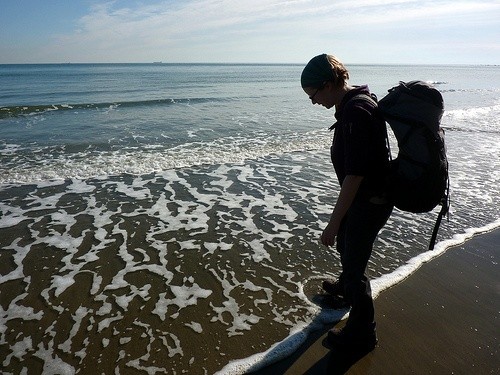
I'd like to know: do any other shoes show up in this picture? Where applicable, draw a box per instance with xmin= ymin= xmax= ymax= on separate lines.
xmin=327 ymin=326 xmax=379 ymax=346
xmin=322 ymin=280 xmax=344 ymax=296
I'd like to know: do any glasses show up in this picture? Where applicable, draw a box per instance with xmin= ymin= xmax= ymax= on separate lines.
xmin=308 ymin=82 xmax=322 ymax=101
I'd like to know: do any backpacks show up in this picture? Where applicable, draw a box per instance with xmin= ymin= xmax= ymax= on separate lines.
xmin=328 ymin=79 xmax=452 ymax=251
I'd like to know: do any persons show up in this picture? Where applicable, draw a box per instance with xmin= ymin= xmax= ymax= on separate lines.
xmin=300 ymin=54 xmax=395 ymax=350
